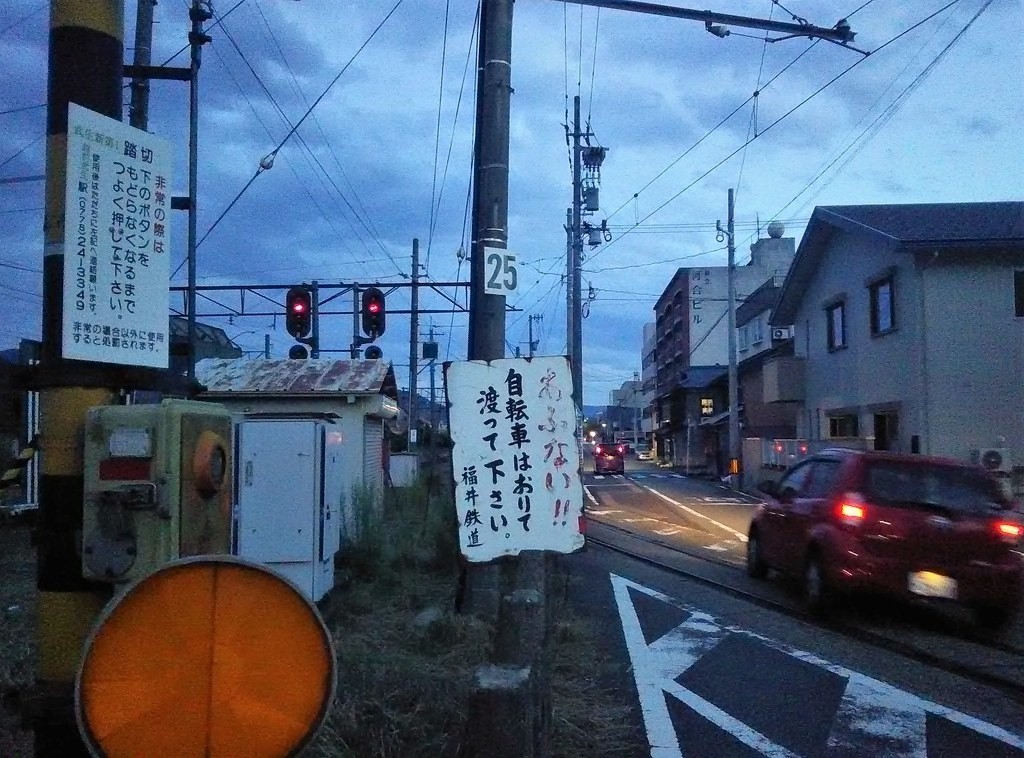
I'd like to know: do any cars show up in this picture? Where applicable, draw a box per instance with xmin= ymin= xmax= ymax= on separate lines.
xmin=591 ymin=442 xmax=625 ymax=475
xmin=745 ymin=445 xmax=1024 ymax=630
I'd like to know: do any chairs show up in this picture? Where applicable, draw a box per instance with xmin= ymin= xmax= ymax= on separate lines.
xmin=892 ymin=479 xmax=928 ymax=502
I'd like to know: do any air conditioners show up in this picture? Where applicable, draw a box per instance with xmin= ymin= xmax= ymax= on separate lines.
xmin=773 ymin=329 xmax=788 ymax=339
xmin=971 ymin=448 xmax=1012 ymax=472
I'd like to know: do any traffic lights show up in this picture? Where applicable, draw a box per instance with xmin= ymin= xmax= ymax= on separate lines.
xmin=285 ymin=287 xmax=311 ymax=337
xmin=362 ymin=288 xmax=386 ymax=338
